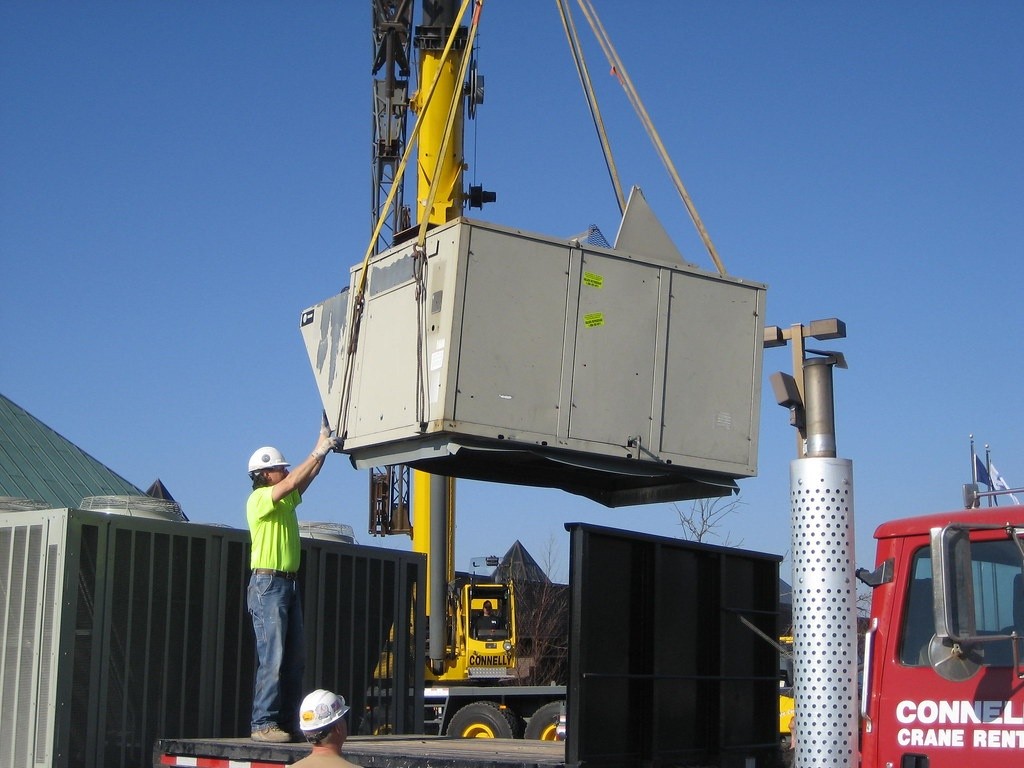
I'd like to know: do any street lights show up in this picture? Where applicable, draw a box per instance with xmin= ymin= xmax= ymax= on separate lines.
xmin=762 ymin=316 xmax=859 ymax=768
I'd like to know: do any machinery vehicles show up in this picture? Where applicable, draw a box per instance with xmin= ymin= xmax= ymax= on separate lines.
xmin=332 ymin=0 xmax=793 ymax=758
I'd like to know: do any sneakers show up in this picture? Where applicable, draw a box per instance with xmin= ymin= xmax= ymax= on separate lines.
xmin=281 ymin=728 xmax=308 ymax=742
xmin=251 ymin=725 xmax=289 ymax=742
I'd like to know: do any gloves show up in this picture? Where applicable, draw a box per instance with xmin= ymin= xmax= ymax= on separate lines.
xmin=312 ymin=437 xmax=337 ymax=463
xmin=320 ymin=409 xmax=331 ymax=438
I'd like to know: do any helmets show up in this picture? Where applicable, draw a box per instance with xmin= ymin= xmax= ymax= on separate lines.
xmin=248 ymin=446 xmax=291 ymax=472
xmin=299 ymin=689 xmax=351 ymax=730
xmin=483 ymin=600 xmax=492 ymax=608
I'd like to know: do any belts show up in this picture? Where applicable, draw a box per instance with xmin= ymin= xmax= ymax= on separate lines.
xmin=253 ymin=568 xmax=298 ymax=579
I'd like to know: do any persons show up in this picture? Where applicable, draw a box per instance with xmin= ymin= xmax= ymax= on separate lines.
xmin=247 ymin=409 xmax=342 ymax=743
xmin=288 ymin=689 xmax=366 ymax=768
xmin=474 ymin=601 xmax=502 ymax=630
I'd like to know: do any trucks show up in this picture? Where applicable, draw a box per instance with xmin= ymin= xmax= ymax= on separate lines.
xmin=154 ymin=485 xmax=1023 ymax=768
